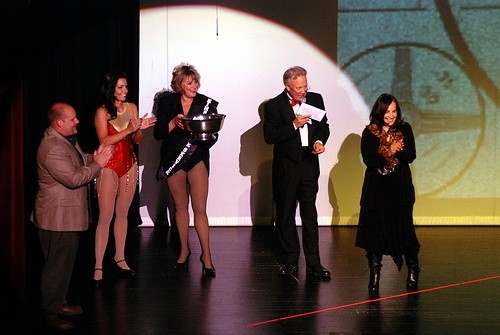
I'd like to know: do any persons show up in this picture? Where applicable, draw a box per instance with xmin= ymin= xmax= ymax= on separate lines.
xmin=30 ymin=102 xmax=114 ymax=330
xmin=263 ymin=67 xmax=331 ymax=278
xmin=153 ymin=62 xmax=219 ymax=277
xmin=93 ymin=71 xmax=157 ymax=287
xmin=354 ymin=93 xmax=422 ymax=294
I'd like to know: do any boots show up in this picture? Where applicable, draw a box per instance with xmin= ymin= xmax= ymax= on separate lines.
xmin=406 ymin=258 xmax=419 ymax=283
xmin=368 ymin=256 xmax=380 ymax=288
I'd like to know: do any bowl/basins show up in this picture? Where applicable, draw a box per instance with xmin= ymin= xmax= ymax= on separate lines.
xmin=178 ymin=113 xmax=226 ymax=141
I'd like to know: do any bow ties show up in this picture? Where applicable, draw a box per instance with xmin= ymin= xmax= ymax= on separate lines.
xmin=291 ymin=98 xmax=302 ymax=106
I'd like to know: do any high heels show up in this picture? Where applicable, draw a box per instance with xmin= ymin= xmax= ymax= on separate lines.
xmin=176 ymin=248 xmax=191 ymax=265
xmin=113 ymin=256 xmax=137 ymax=275
xmin=93 ymin=265 xmax=104 ymax=286
xmin=200 ymin=254 xmax=216 ymax=274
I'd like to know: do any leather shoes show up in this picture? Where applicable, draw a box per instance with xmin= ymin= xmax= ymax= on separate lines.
xmin=308 ymin=265 xmax=330 ymax=277
xmin=47 ymin=304 xmax=83 ymax=315
xmin=278 ymin=263 xmax=297 ymax=275
xmin=43 ymin=315 xmax=73 ymax=330
